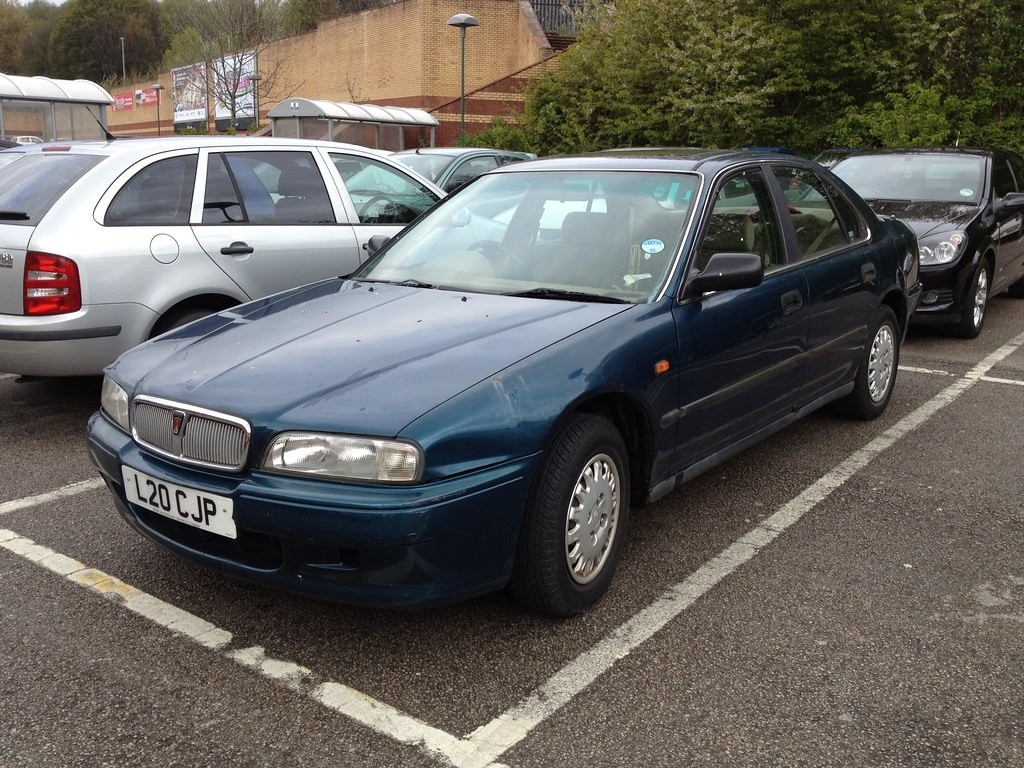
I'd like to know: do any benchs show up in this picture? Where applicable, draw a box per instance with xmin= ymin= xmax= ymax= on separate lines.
xmin=616 ymin=197 xmax=846 ymax=261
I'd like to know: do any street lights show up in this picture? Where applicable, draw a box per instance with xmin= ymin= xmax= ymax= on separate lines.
xmin=151 ymin=84 xmax=165 ymax=137
xmin=248 ymin=74 xmax=263 ymax=127
xmin=447 ymin=13 xmax=480 ymax=146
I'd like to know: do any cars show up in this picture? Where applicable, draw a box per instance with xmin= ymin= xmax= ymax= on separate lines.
xmin=10 ymin=136 xmax=43 ymax=146
xmin=85 ymin=146 xmax=920 ymax=618
xmin=790 ymin=146 xmax=1024 ymax=338
xmin=388 ymin=145 xmax=537 ymax=192
xmin=728 ymin=146 xmax=791 ymax=194
xmin=791 ymin=149 xmax=868 ymax=196
xmin=493 ymin=148 xmax=766 ymax=232
xmin=0 ymin=133 xmax=511 ymax=397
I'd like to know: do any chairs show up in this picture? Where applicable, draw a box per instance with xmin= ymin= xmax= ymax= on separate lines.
xmin=537 ymin=208 xmax=611 ymax=291
xmin=270 ymin=168 xmax=340 ymax=223
xmin=886 ymin=169 xmax=928 ymax=202
xmin=943 ymin=171 xmax=982 ymax=201
xmin=685 ymin=212 xmax=756 ymax=275
